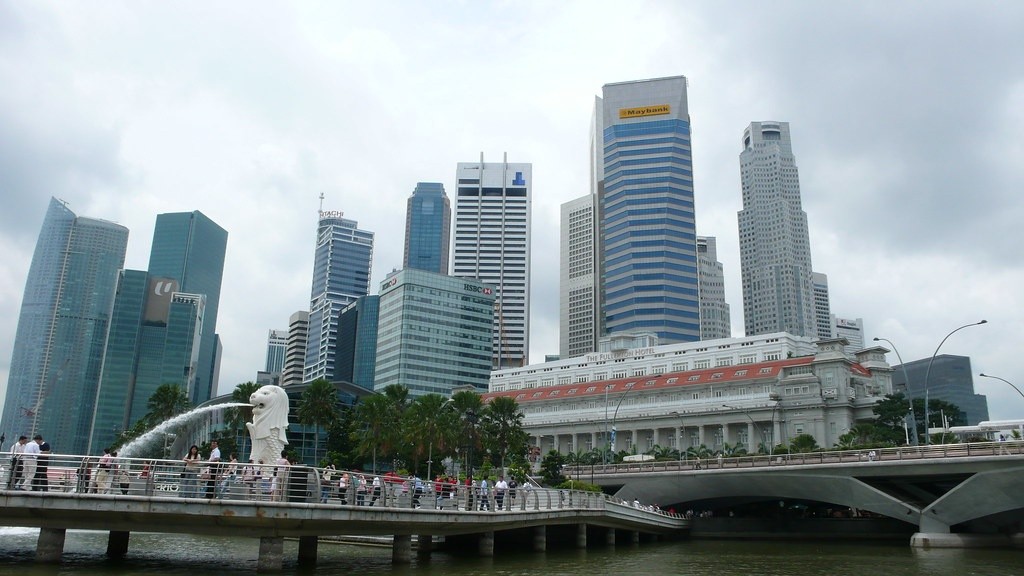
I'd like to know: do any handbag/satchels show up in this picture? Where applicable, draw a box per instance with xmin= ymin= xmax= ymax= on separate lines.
xmin=200 ymin=471 xmax=211 ymax=483
xmin=227 ymin=469 xmax=234 ymax=474
xmin=181 ymin=471 xmax=185 ymax=478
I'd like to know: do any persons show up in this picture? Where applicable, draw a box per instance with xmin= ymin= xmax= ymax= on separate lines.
xmin=523 ymin=479 xmax=531 ymax=493
xmin=320 ymin=461 xmax=336 ymax=504
xmin=216 ymin=452 xmax=238 ymax=499
xmin=693 ymin=455 xmax=702 ymax=469
xmin=201 ymin=439 xmax=221 ymax=499
xmin=403 ymin=473 xmax=518 ymax=511
xmin=368 ymin=476 xmax=380 ymax=506
xmin=178 ymin=445 xmax=201 ymax=498
xmin=716 ymin=451 xmax=722 ymax=468
xmin=339 ymin=468 xmax=367 ymax=506
xmin=80 ymin=448 xmax=130 ymax=494
xmin=7 ymin=434 xmax=50 ymax=492
xmin=241 ymin=459 xmax=264 ymax=501
xmin=140 ymin=461 xmax=150 ymax=479
xmin=269 ymin=450 xmax=291 ymax=501
xmin=620 ymin=498 xmax=713 ymax=519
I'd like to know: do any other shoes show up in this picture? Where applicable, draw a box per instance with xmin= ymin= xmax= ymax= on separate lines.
xmin=103 ymin=489 xmax=109 ymax=494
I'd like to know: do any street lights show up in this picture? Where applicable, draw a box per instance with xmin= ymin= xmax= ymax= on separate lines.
xmin=723 ymin=404 xmax=765 ymax=457
xmin=611 ymin=374 xmax=662 ymax=463
xmin=426 ymin=397 xmax=456 ymax=482
xmin=874 ymin=338 xmax=919 ymax=448
xmin=602 ymin=385 xmax=608 ymax=470
xmin=768 ymin=400 xmax=780 ymax=467
xmin=925 ymin=320 xmax=987 ymax=445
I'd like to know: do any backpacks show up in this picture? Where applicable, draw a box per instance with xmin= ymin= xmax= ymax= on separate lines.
xmin=526 ymin=482 xmax=531 ymax=492
